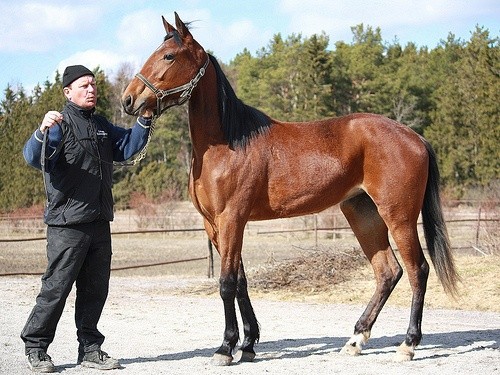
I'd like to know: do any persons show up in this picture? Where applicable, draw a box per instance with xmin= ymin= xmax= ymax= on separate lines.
xmin=20 ymin=65 xmax=152 ymax=373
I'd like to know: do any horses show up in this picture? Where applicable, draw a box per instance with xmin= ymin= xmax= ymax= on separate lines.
xmin=122 ymin=12 xmax=465 ymax=366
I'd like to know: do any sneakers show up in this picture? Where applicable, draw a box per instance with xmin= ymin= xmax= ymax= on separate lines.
xmin=27 ymin=352 xmax=55 ymax=372
xmin=77 ymin=350 xmax=121 ymax=369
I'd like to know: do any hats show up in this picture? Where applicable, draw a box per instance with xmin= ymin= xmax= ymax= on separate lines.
xmin=63 ymin=64 xmax=95 ymax=88
